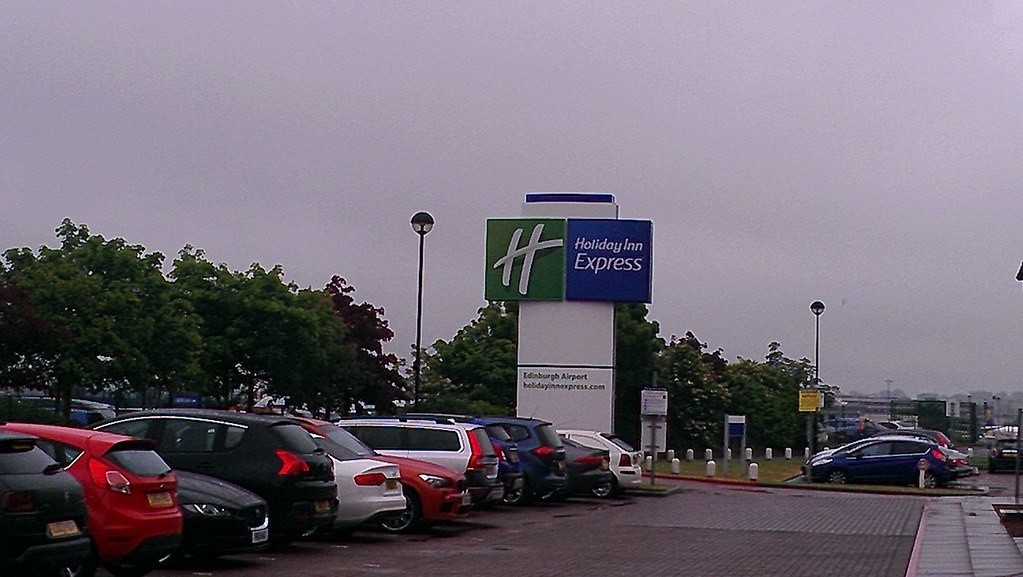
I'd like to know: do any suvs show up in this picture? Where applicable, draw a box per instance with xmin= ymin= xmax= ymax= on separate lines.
xmin=88 ymin=405 xmax=341 ymax=549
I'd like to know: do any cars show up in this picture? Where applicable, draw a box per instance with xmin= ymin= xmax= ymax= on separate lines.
xmin=295 ymin=416 xmax=473 ymax=535
xmin=327 ymin=412 xmax=643 ymax=512
xmin=1 ymin=426 xmax=91 ymax=577
xmin=156 ymin=469 xmax=272 ymax=565
xmin=799 ymin=417 xmax=973 ymax=490
xmin=986 ymin=436 xmax=1023 ymax=475
xmin=0 ymin=418 xmax=186 ymax=577
xmin=174 ymin=416 xmax=409 ymax=530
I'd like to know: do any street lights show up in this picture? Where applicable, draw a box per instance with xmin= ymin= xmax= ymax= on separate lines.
xmin=408 ymin=210 xmax=434 ymax=414
xmin=808 ymin=299 xmax=826 ymax=387
